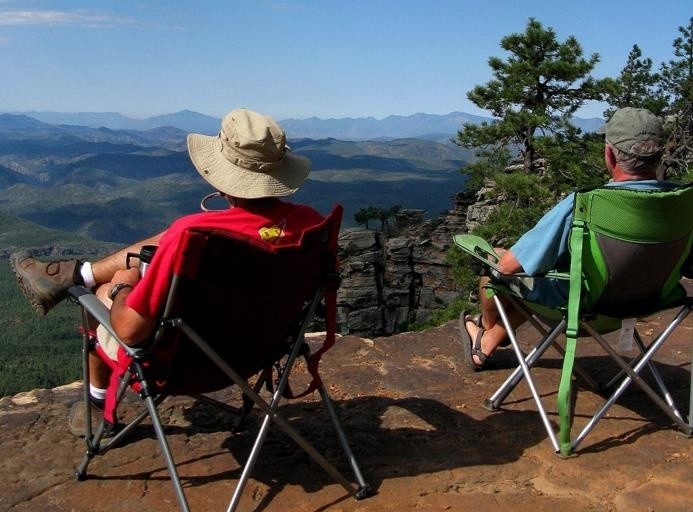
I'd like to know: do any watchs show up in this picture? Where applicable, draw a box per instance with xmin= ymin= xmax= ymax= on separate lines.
xmin=111 ymin=283 xmax=133 ymax=300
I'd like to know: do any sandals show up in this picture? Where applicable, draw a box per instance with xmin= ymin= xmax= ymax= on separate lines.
xmin=460 ymin=309 xmax=516 ymax=371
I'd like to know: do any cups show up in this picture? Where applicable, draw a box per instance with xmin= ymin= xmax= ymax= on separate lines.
xmin=125 ymin=244 xmax=155 ymax=285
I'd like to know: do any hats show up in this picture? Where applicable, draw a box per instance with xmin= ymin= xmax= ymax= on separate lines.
xmin=187 ymin=108 xmax=311 ymax=199
xmin=596 ymin=106 xmax=665 ymax=160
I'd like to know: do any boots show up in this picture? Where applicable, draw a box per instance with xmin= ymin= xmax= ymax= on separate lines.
xmin=68 ymin=395 xmax=118 ymax=439
xmin=11 ymin=249 xmax=76 ymax=315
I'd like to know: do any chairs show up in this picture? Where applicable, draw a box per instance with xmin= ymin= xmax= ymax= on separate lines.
xmin=441 ymin=176 xmax=692 ymax=464
xmin=61 ymin=197 xmax=379 ymax=511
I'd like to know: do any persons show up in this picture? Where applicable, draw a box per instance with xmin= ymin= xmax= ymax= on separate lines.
xmin=9 ymin=108 xmax=326 ymax=437
xmin=459 ymin=107 xmax=693 ymax=372
